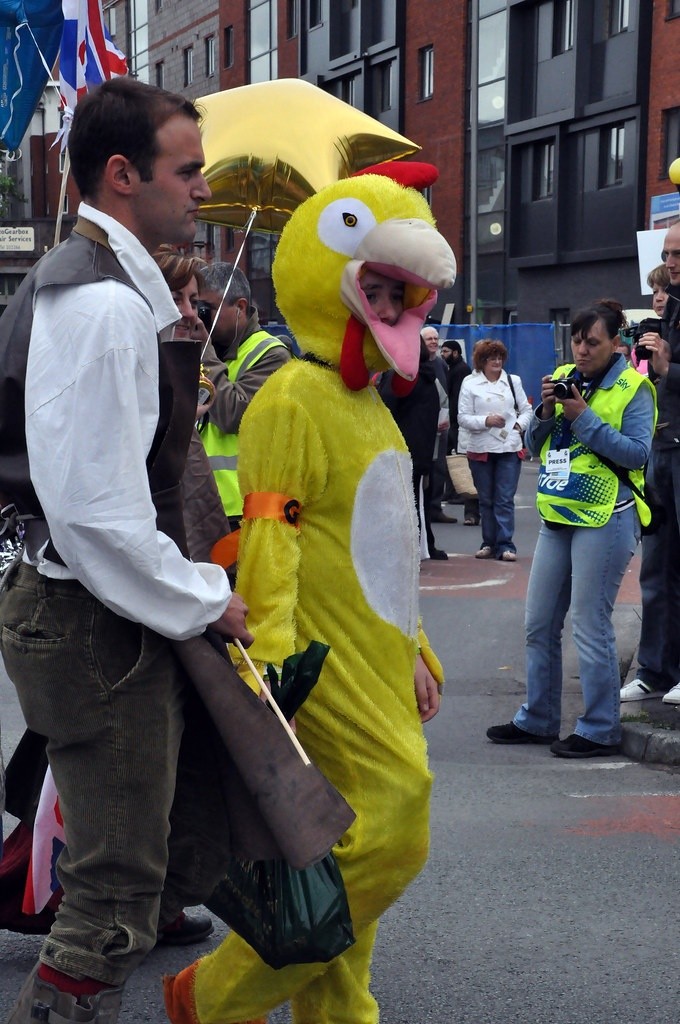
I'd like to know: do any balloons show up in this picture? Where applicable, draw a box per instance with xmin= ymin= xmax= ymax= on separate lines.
xmin=187 ymin=77 xmax=421 ymax=233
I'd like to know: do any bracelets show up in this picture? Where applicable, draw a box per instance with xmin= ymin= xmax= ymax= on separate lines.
xmin=416 ymin=649 xmax=421 ymax=654
xmin=264 ymin=674 xmax=281 ymax=680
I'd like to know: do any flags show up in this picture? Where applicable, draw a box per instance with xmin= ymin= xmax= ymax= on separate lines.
xmin=0 ymin=0 xmax=130 ymax=152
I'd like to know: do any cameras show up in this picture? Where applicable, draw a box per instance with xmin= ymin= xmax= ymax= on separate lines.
xmin=195 ymin=302 xmax=211 ymax=331
xmin=550 ymin=378 xmax=582 ymax=399
xmin=623 ymin=318 xmax=662 ymax=360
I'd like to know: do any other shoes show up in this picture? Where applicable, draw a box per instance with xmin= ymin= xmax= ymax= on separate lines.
xmin=433 ymin=512 xmax=457 ymax=523
xmin=449 ymin=494 xmax=468 ymax=505
xmin=463 ymin=517 xmax=477 ymax=525
xmin=429 ymin=545 xmax=448 ymax=559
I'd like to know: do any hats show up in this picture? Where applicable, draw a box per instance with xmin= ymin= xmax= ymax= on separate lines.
xmin=442 ymin=341 xmax=462 ymax=354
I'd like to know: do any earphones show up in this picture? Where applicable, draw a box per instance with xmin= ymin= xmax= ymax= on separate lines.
xmin=237 ymin=307 xmax=241 ymax=317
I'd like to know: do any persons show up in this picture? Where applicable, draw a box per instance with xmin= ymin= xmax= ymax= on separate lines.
xmin=372 ymin=327 xmax=479 ymax=559
xmin=457 ymin=339 xmax=534 ymax=562
xmin=486 ymin=301 xmax=659 ymax=757
xmin=161 ymin=161 xmax=457 ymax=1024
xmin=150 ymin=247 xmax=293 ymax=945
xmin=616 ymin=223 xmax=680 ymax=704
xmin=0 ymin=77 xmax=358 ymax=1024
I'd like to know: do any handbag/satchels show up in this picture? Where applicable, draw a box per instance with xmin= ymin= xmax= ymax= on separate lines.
xmin=642 ymin=482 xmax=667 ymax=536
xmin=162 ymin=641 xmax=356 ymax=968
xmin=446 ymin=449 xmax=478 ymax=495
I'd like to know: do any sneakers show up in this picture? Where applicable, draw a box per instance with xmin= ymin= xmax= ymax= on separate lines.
xmin=485 ymin=722 xmax=560 ymax=744
xmin=157 ymin=908 xmax=214 ymax=948
xmin=502 ymin=551 xmax=517 ymax=561
xmin=475 ymin=546 xmax=494 ymax=558
xmin=662 ymin=682 xmax=680 ymax=704
xmin=550 ymin=734 xmax=621 ymax=758
xmin=620 ymin=678 xmax=666 ymax=702
